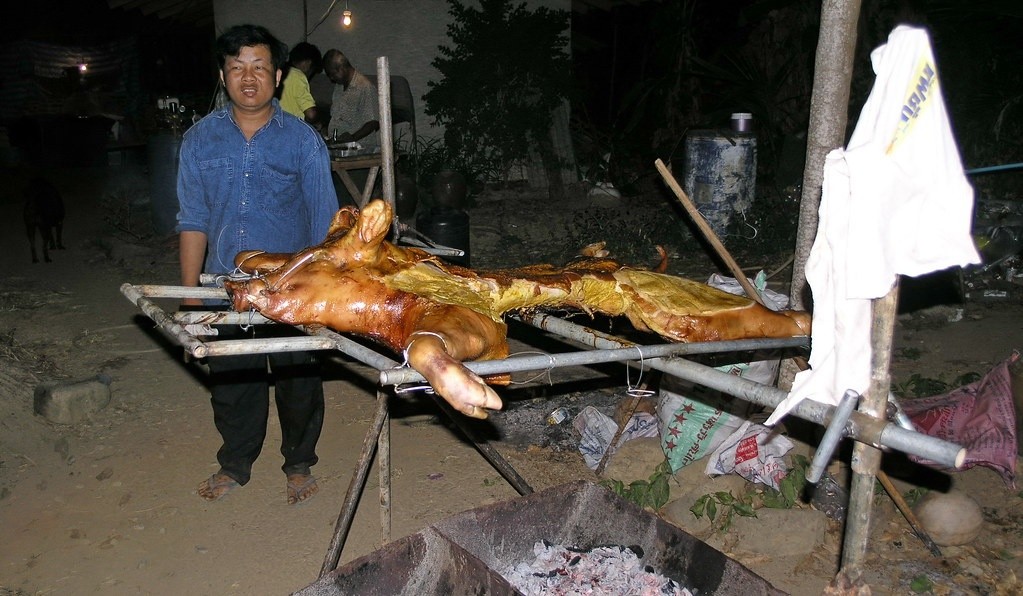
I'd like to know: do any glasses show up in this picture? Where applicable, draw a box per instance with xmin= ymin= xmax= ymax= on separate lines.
xmin=327 ymin=65 xmax=341 ymax=78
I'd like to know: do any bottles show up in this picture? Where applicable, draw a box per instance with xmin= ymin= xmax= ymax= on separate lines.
xmin=416 ymin=207 xmax=470 ymax=268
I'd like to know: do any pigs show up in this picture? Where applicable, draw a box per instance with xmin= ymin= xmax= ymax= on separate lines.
xmin=224 ymin=199 xmax=813 ymax=421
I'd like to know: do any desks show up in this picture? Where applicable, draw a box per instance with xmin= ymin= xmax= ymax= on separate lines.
xmin=328 ymin=143 xmax=398 ymax=208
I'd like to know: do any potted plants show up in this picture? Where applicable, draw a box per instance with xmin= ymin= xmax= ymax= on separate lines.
xmin=391 ymin=135 xmax=475 ymax=266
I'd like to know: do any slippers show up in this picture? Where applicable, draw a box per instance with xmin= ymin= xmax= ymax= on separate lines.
xmin=287 ymin=473 xmax=319 ymax=504
xmin=198 ymin=473 xmax=237 ymax=502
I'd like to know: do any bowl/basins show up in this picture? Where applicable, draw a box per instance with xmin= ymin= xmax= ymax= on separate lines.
xmin=731 ymin=113 xmax=755 ymax=132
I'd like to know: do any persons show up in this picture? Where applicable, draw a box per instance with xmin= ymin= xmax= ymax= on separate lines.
xmin=215 ymin=42 xmax=383 ymax=202
xmin=176 ymin=24 xmax=339 ymax=506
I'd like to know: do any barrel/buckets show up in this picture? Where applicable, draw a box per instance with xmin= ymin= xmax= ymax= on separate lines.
xmin=147 ymin=136 xmax=182 ymax=233
xmin=685 ymin=128 xmax=758 ymax=235
